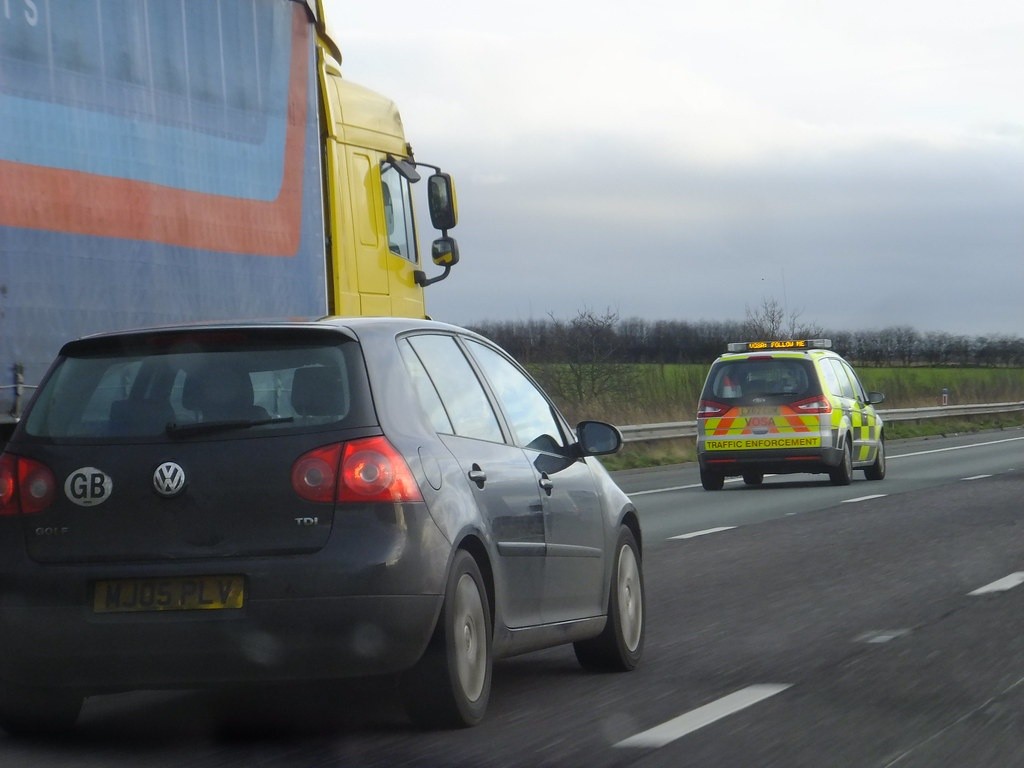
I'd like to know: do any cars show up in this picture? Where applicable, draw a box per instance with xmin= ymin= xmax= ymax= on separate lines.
xmin=0 ymin=317 xmax=645 ymax=729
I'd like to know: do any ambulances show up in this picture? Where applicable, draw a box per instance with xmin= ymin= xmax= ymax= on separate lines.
xmin=696 ymin=339 xmax=886 ymax=491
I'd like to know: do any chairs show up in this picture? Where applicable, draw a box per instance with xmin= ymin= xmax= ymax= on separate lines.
xmin=107 ymin=401 xmax=175 ymax=428
xmin=182 ymin=359 xmax=272 ymax=422
xmin=742 ymin=380 xmax=770 ymax=397
xmin=291 ymin=366 xmax=346 ymax=419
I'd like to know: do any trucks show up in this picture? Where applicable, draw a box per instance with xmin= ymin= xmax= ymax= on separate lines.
xmin=1 ymin=0 xmax=459 ymax=425
